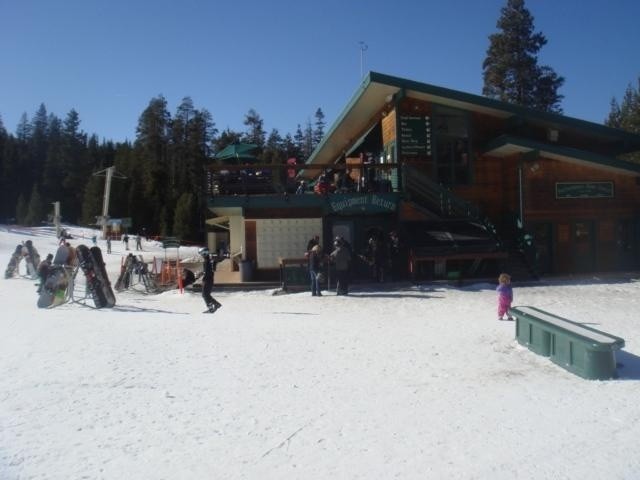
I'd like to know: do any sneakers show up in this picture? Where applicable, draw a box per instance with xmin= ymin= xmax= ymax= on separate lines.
xmin=202 ymin=299 xmax=222 ymax=314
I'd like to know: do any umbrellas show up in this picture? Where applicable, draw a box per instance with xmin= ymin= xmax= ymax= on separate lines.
xmin=219 ymin=152 xmax=256 ymax=161
xmin=215 ymin=138 xmax=259 ymax=164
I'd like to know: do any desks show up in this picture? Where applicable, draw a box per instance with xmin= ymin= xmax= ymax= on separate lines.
xmin=279 ymin=257 xmax=331 ymax=291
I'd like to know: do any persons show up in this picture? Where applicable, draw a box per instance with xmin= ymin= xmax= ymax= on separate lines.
xmin=242 ymin=163 xmax=255 ymax=176
xmin=57 ymin=228 xmax=67 ymax=247
xmin=123 ymin=231 xmax=130 ymax=251
xmin=176 ymin=268 xmax=195 ymax=289
xmin=34 ymin=253 xmax=54 ymax=293
xmin=3 ymin=245 xmax=26 ymax=279
xmin=135 ymin=233 xmax=143 ymax=251
xmin=256 ymin=159 xmax=272 ymax=176
xmin=286 ymin=152 xmax=379 ymax=194
xmin=107 ymin=237 xmax=112 ymax=254
xmin=494 ymin=272 xmax=515 ymax=320
xmin=198 ymin=247 xmax=221 ymax=314
xmin=22 ymin=240 xmax=40 ymax=275
xmin=91 ymin=235 xmax=98 ymax=248
xmin=303 ymin=229 xmax=404 ymax=296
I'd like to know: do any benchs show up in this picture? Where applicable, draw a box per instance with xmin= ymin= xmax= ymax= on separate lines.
xmin=509 ymin=304 xmax=626 ymax=380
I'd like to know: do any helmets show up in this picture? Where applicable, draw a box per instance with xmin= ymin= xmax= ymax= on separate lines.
xmin=198 ymin=247 xmax=209 ymax=257
